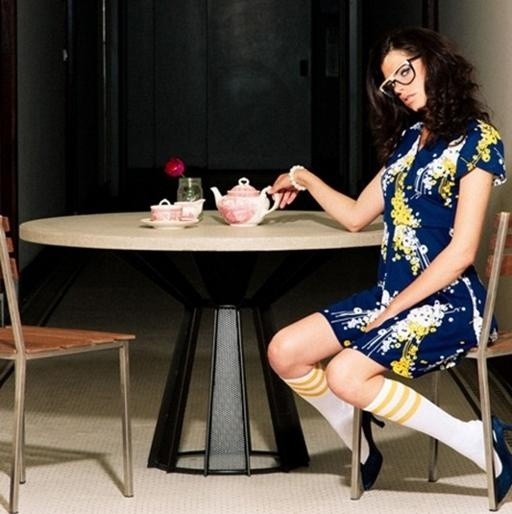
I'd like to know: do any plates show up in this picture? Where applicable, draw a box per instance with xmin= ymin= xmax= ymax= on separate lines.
xmin=142 ymin=218 xmax=198 ymax=231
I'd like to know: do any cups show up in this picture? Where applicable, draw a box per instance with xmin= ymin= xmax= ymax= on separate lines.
xmin=174 ymin=199 xmax=205 ymax=220
xmin=150 ymin=197 xmax=183 ymax=221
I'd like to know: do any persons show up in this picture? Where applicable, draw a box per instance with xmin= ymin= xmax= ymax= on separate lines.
xmin=267 ymin=27 xmax=512 ymax=510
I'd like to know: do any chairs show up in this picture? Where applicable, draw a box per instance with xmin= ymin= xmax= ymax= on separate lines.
xmin=351 ymin=210 xmax=512 ymax=511
xmin=1 ymin=216 xmax=136 ymax=514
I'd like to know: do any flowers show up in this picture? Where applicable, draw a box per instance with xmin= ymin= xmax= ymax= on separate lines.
xmin=165 ymin=159 xmax=196 ymax=198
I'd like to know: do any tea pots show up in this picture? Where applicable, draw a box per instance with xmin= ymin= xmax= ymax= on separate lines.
xmin=211 ymin=177 xmax=282 ymax=227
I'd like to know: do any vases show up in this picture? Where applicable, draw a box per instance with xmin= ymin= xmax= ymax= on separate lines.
xmin=177 ymin=177 xmax=203 ymax=221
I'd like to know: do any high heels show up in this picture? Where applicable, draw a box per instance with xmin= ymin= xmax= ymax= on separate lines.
xmin=359 ymin=410 xmax=385 ymax=492
xmin=488 ymin=414 xmax=511 ymax=509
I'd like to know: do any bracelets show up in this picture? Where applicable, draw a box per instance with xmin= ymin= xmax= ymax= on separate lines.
xmin=290 ymin=164 xmax=306 ymax=191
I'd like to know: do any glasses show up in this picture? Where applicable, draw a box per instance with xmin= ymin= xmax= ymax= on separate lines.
xmin=380 ymin=55 xmax=422 ymax=97
